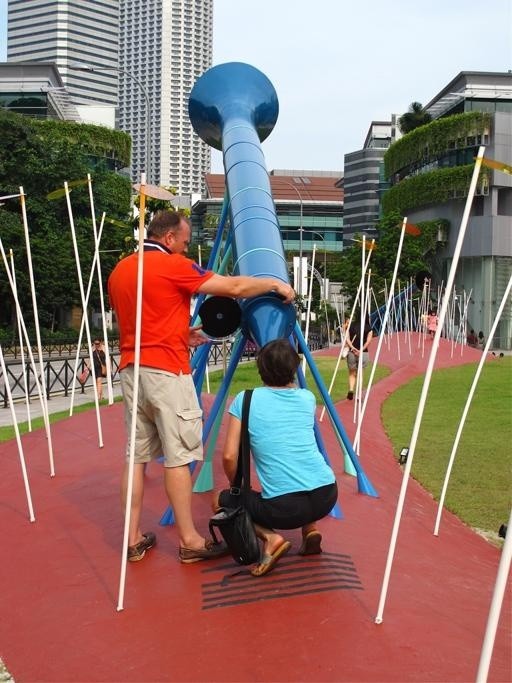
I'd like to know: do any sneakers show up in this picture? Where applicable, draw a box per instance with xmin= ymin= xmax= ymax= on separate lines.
xmin=178 ymin=536 xmax=229 ymax=564
xmin=127 ymin=530 xmax=156 ymax=562
xmin=347 ymin=391 xmax=353 ymax=400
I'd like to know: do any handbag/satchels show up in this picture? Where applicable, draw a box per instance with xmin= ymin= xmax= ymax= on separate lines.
xmin=342 ymin=347 xmax=350 ymax=359
xmin=101 ymin=366 xmax=107 ymax=375
xmin=79 ymin=368 xmax=90 ymax=384
xmin=210 ymin=506 xmax=259 ymax=564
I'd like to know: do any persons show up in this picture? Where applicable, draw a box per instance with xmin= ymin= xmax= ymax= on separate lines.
xmin=210 ymin=337 xmax=338 ymax=576
xmin=477 ymin=329 xmax=486 ymax=350
xmin=85 ymin=336 xmax=106 ymax=400
xmin=105 ymin=209 xmax=297 ymax=563
xmin=344 ymin=306 xmax=373 ymax=402
xmin=428 ymin=308 xmax=440 ymax=340
xmin=468 ymin=329 xmax=478 ymax=347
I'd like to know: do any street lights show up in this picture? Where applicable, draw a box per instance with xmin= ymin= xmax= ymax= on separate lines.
xmin=69 ymin=63 xmax=151 ymax=184
xmin=298 ymin=229 xmax=326 ymax=300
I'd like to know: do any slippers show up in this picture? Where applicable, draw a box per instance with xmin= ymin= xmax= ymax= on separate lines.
xmin=250 ymin=540 xmax=292 ymax=577
xmin=298 ymin=528 xmax=323 ymax=556
